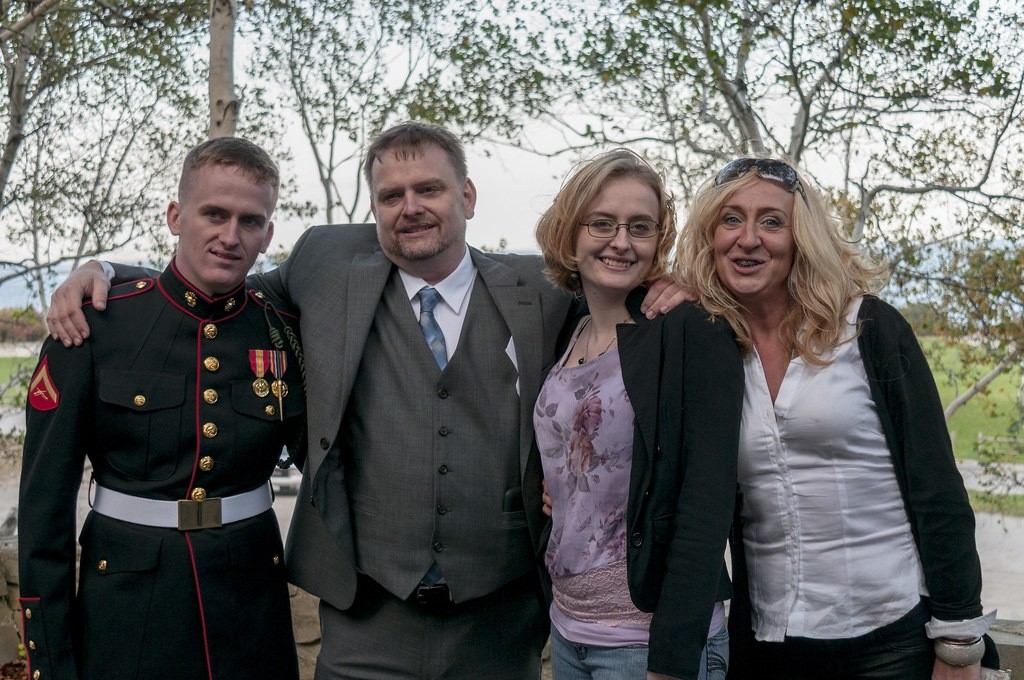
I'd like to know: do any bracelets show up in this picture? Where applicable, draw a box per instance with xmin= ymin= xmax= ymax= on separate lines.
xmin=936 ymin=636 xmax=985 ymax=665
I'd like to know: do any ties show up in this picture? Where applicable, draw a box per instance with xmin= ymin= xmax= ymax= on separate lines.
xmin=417 ymin=287 xmax=448 ymax=372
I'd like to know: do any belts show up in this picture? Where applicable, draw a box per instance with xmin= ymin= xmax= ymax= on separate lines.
xmin=91 ymin=479 xmax=272 ymax=530
xmin=356 ymin=568 xmax=510 ymax=607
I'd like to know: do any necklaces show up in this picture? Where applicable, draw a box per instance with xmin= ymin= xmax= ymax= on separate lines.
xmin=581 ymin=317 xmax=631 ymax=364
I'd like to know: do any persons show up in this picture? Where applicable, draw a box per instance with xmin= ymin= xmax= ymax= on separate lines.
xmin=532 ymin=148 xmax=745 ymax=680
xmin=47 ymin=122 xmax=703 ymax=680
xmin=17 ymin=135 xmax=308 ymax=680
xmin=542 ymin=159 xmax=997 ymax=680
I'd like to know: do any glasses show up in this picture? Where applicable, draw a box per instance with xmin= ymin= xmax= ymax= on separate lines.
xmin=574 ymin=218 xmax=662 ymax=238
xmin=714 ymin=159 xmax=810 ymax=210
